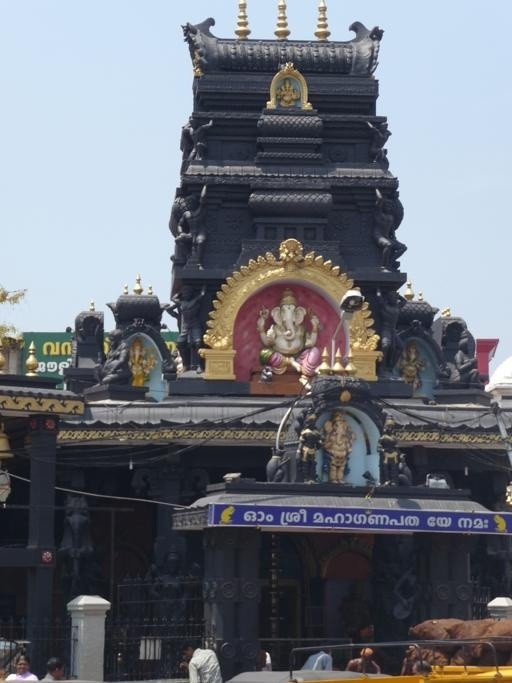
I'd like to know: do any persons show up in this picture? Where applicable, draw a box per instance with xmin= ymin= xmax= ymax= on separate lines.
xmin=453 ymin=336 xmax=481 ymax=383
xmin=412 ymin=660 xmax=432 ymax=675
xmin=178 ymin=639 xmax=223 ymax=682
xmin=168 ymin=116 xmax=213 ymax=374
xmin=376 ymin=418 xmax=400 ymax=485
xmin=99 ymin=326 xmax=129 ymax=384
xmin=3 ymin=654 xmax=41 ymax=682
xmin=345 ymin=647 xmax=380 ymax=673
xmin=300 ymin=649 xmax=333 ymax=671
xmin=37 ymin=654 xmax=66 ymax=682
xmin=362 ymin=117 xmax=409 ymax=381
xmin=294 ymin=411 xmax=323 ymax=488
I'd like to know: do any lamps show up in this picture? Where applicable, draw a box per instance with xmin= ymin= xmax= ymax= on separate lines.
xmin=330 ymin=289 xmax=363 ymax=372
